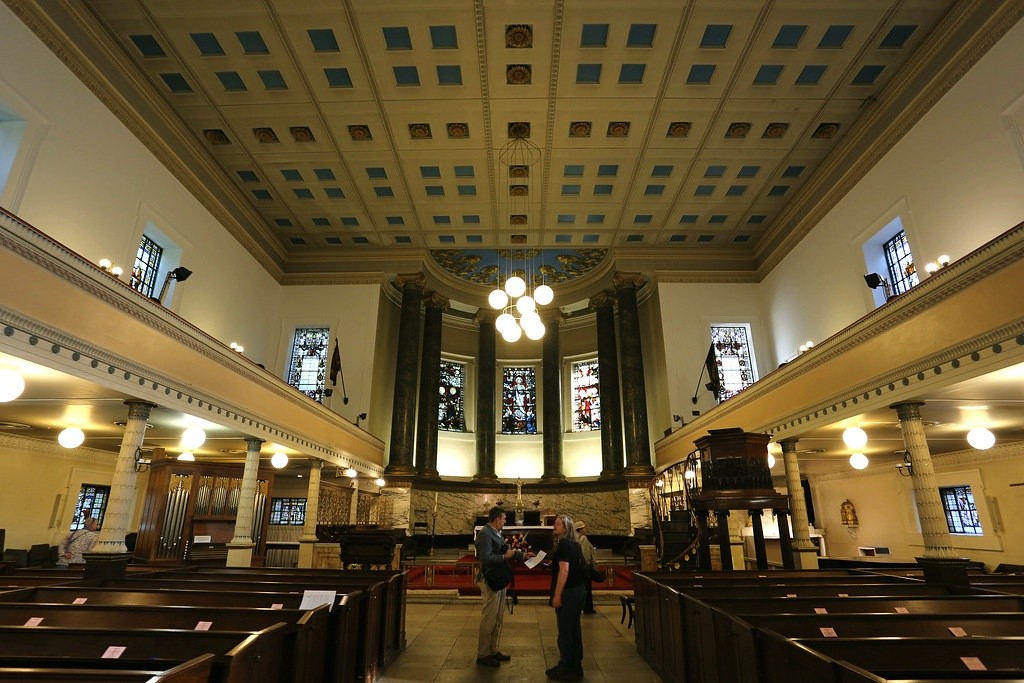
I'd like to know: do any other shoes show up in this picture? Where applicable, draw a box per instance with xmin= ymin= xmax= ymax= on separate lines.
xmin=476 ymin=655 xmax=500 ymax=667
xmin=545 ymin=660 xmax=584 ymax=680
xmin=492 ymin=652 xmax=511 ymax=661
xmin=583 ymin=610 xmax=596 ymax=614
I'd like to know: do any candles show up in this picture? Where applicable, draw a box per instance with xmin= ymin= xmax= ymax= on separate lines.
xmin=434 ymin=492 xmax=438 ymax=512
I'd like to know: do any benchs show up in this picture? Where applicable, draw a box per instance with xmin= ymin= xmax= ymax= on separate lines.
xmin=0 ymin=544 xmax=411 ymax=683
xmin=632 ymin=561 xmax=1024 ymax=683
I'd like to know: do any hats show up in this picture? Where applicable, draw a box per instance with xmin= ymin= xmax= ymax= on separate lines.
xmin=574 ymin=521 xmax=585 ymax=529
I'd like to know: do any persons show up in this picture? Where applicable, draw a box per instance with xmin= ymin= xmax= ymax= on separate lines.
xmin=56 ymin=518 xmax=99 ymax=565
xmin=846 ymin=506 xmax=854 ymax=525
xmin=574 ymin=521 xmax=596 ymax=615
xmin=542 ymin=515 xmax=588 ymax=681
xmin=504 ymin=377 xmax=531 ymax=421
xmin=475 ymin=506 xmax=537 ymax=667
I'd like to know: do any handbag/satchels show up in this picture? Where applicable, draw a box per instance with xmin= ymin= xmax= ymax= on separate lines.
xmin=592 ymin=566 xmax=607 ymax=583
xmin=485 ymin=562 xmax=512 ymax=592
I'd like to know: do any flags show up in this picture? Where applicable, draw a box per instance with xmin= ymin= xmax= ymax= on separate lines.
xmin=330 ymin=337 xmax=341 ymax=386
xmin=706 ymin=342 xmax=720 ymax=401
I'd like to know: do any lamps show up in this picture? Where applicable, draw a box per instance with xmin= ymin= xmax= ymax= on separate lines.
xmin=895 ymin=448 xmax=914 ymax=477
xmin=673 ymin=414 xmax=684 ymax=426
xmin=800 ymin=340 xmax=813 ymax=354
xmin=488 ymin=129 xmax=554 ymax=343
xmin=360 ymin=413 xmax=367 ymax=420
xmin=231 ymin=342 xmax=244 ymax=355
xmin=100 ymin=258 xmax=123 ymax=278
xmin=169 ymin=267 xmax=193 ymax=282
xmin=863 ymin=272 xmax=885 ymax=290
xmin=925 ymin=255 xmax=950 ymax=274
